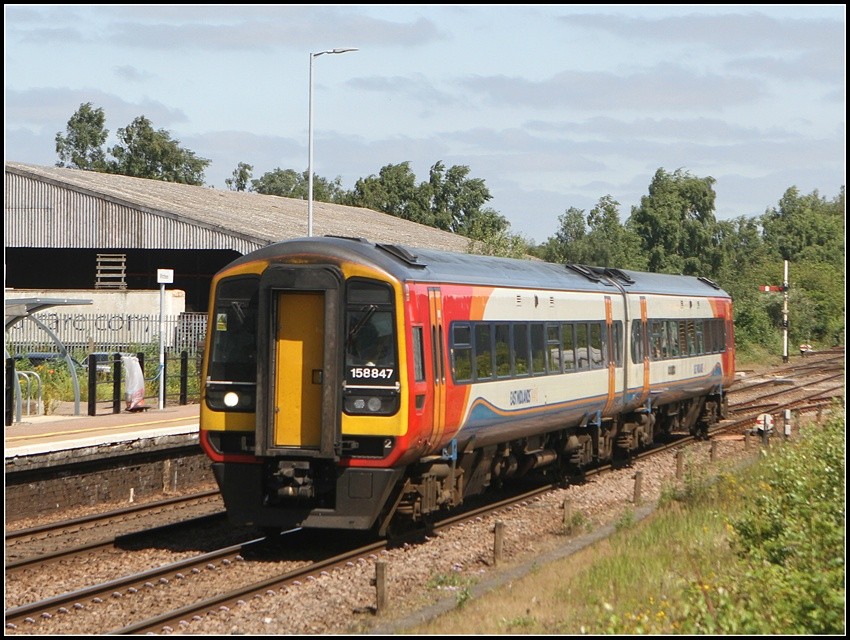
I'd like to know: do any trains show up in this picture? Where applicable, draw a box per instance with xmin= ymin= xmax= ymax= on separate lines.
xmin=197 ymin=231 xmax=736 ymax=538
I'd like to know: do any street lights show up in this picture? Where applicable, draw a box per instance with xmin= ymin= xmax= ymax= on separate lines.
xmin=306 ymin=45 xmax=360 ymax=236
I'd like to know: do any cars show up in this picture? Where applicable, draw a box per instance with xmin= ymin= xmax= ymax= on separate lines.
xmin=11 ymin=351 xmax=82 ymax=373
xmin=79 ymin=352 xmax=137 ymax=375
